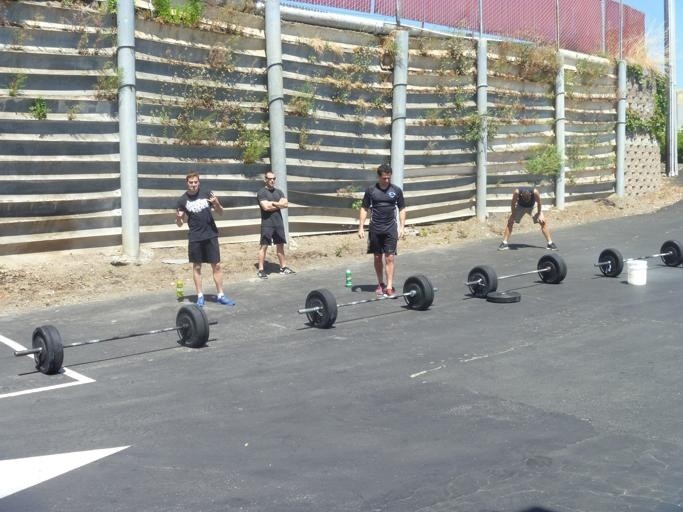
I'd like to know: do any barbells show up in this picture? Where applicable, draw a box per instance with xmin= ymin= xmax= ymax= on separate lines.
xmin=594 ymin=240 xmax=682 ymax=277
xmin=466 ymin=254 xmax=567 ymax=298
xmin=14 ymin=306 xmax=218 ymax=377
xmin=299 ymin=276 xmax=438 ymax=329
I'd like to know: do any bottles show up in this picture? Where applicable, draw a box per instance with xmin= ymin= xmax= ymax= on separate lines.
xmin=175 ymin=275 xmax=184 ymax=301
xmin=345 ymin=268 xmax=352 ymax=288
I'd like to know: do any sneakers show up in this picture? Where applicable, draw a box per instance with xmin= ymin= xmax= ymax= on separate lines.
xmin=497 ymin=241 xmax=510 ymax=251
xmin=383 ymin=288 xmax=398 ymax=299
xmin=279 ymin=266 xmax=296 ymax=275
xmin=546 ymin=242 xmax=558 ymax=251
xmin=257 ymin=269 xmax=268 ymax=279
xmin=194 ymin=295 xmax=206 ymax=308
xmin=375 ymin=282 xmax=384 ymax=295
xmin=216 ymin=294 xmax=235 ymax=306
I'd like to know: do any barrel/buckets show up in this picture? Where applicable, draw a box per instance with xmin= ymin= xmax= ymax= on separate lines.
xmin=626 ymin=259 xmax=648 ymax=286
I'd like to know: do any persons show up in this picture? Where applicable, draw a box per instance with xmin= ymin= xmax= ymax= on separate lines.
xmin=257 ymin=172 xmax=297 ymax=280
xmin=497 ymin=186 xmax=560 ymax=252
xmin=175 ymin=173 xmax=236 ymax=306
xmin=358 ymin=165 xmax=406 ymax=300
